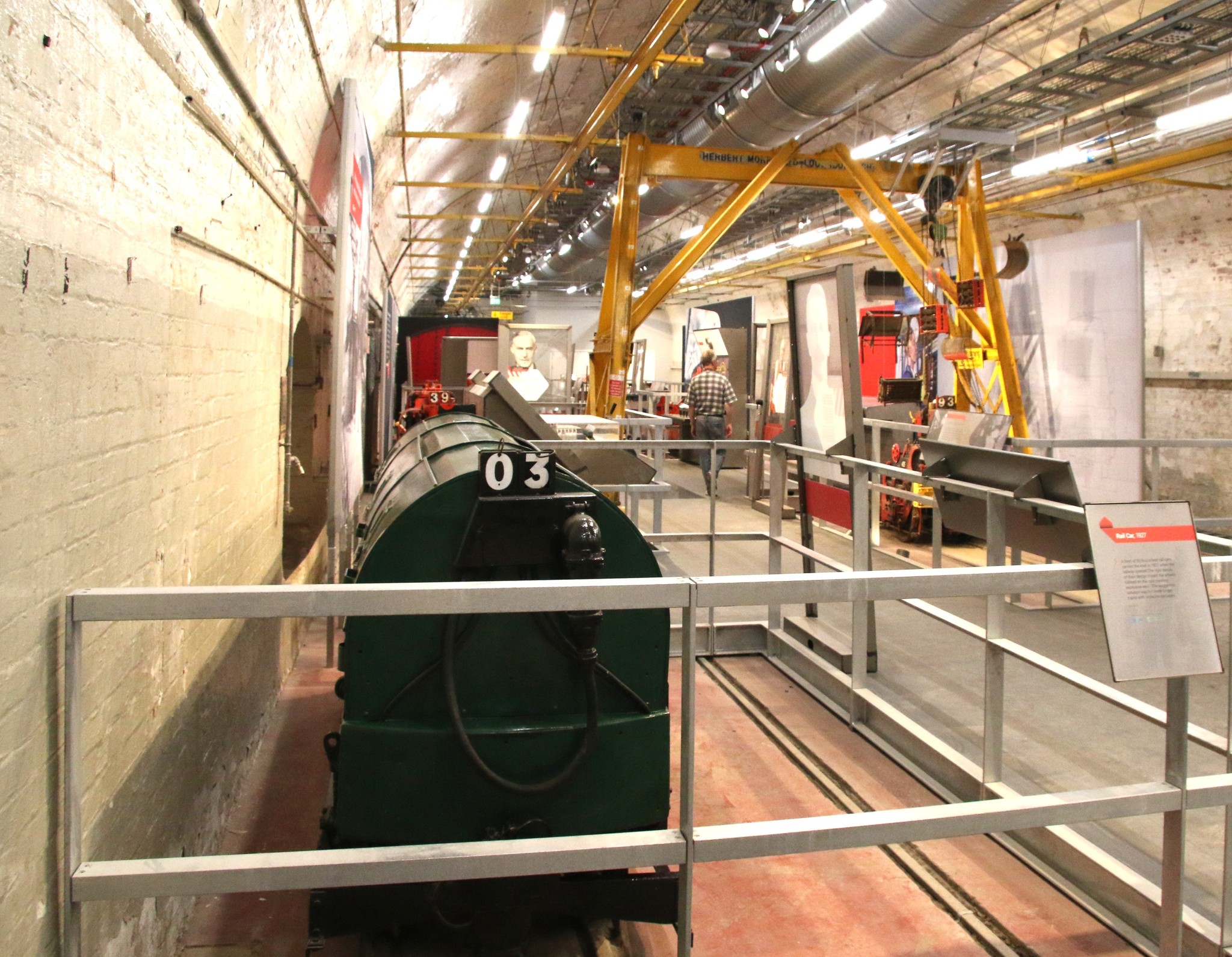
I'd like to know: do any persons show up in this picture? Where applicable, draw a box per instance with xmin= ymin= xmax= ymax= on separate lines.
xmin=511 ymin=330 xmax=536 ymax=369
xmin=688 ymin=349 xmax=738 ymax=496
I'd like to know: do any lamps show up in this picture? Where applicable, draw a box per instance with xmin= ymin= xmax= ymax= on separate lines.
xmin=442 ymin=0 xmax=1231 ymax=302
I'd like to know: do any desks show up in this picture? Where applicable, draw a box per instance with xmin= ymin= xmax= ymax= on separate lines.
xmin=539 ymin=414 xmax=620 ymax=441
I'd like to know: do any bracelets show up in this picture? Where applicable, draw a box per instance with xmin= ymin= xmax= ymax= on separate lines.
xmin=690 ymin=422 xmax=695 ymax=426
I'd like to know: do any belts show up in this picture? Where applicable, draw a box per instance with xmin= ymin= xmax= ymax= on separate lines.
xmin=697 ymin=412 xmax=723 ymax=418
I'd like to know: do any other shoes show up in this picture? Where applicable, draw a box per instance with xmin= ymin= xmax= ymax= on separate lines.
xmin=705 ymin=491 xmax=717 ymax=496
xmin=706 ymin=471 xmax=717 ymax=490
